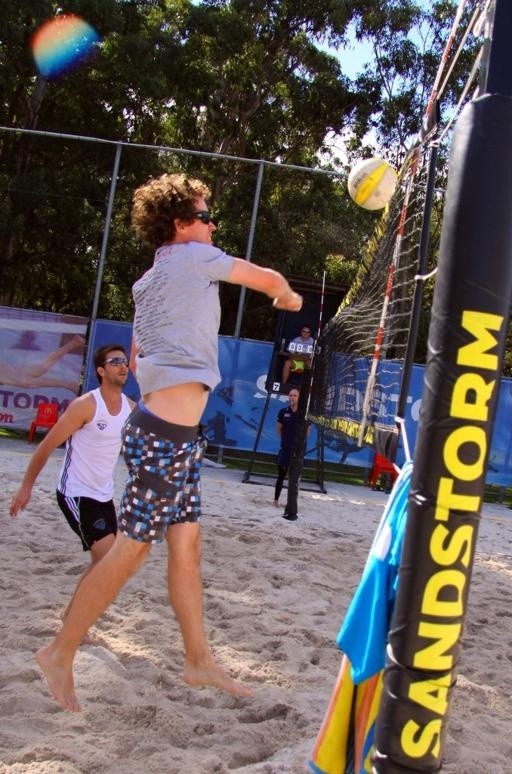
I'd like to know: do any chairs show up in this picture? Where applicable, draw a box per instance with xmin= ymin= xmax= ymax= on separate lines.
xmin=28 ymin=403 xmax=58 ymax=441
xmin=367 ymin=453 xmax=398 ymax=487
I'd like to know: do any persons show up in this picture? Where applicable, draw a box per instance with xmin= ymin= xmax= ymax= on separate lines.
xmin=10 ymin=345 xmax=138 ymax=644
xmin=274 ymin=389 xmax=311 ymax=507
xmin=0 ymin=335 xmax=86 ymax=398
xmin=35 ymin=174 xmax=303 ymax=712
xmin=282 ymin=326 xmax=314 ymax=384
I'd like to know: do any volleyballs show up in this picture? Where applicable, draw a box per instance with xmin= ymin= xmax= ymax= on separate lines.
xmin=347 ymin=159 xmax=398 ymax=209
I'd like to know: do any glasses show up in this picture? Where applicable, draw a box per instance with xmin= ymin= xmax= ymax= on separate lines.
xmin=180 ymin=211 xmax=212 ymax=224
xmin=100 ymin=358 xmax=129 ymax=366
